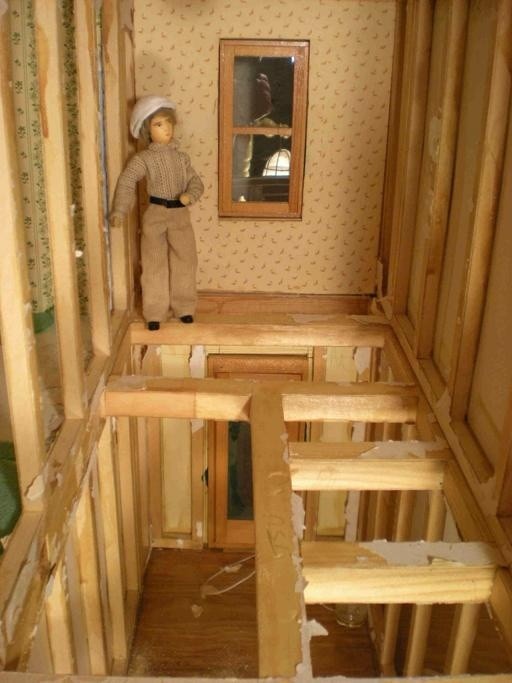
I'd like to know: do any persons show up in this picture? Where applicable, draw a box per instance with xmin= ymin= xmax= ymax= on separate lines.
xmin=104 ymin=92 xmax=205 ymax=330
